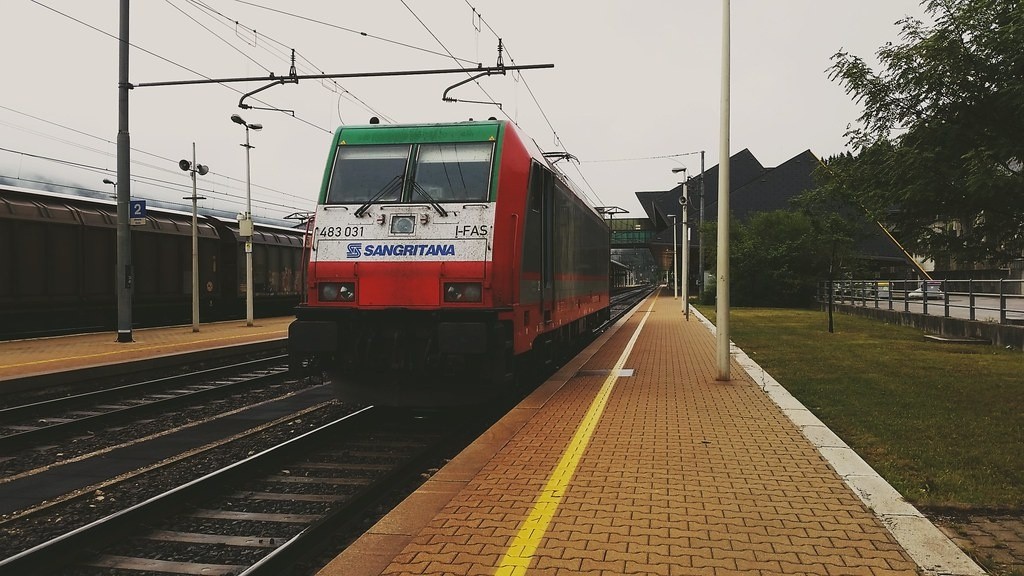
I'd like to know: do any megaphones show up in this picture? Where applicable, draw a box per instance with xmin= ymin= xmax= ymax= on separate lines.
xmin=197 ymin=164 xmax=209 ymax=175
xmin=679 ymin=196 xmax=687 ymax=205
xmin=179 ymin=159 xmax=192 ymax=171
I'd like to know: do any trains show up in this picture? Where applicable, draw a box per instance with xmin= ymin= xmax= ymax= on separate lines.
xmin=288 ymin=117 xmax=611 ymax=404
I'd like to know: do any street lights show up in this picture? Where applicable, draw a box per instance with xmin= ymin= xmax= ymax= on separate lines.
xmin=231 ymin=113 xmax=263 ymax=327
